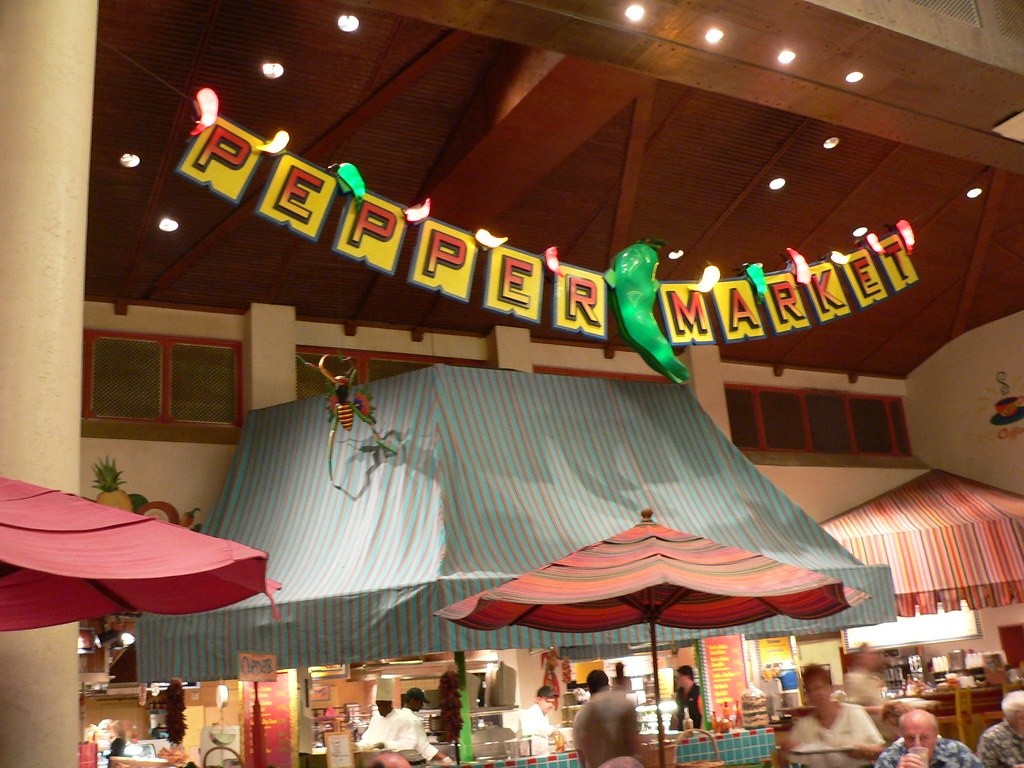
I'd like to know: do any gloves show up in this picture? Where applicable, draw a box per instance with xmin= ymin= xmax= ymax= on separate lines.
xmin=443 ymin=756 xmax=453 ymax=764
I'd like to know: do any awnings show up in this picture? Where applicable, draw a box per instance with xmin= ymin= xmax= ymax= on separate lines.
xmin=819 ymin=469 xmax=1024 ymax=617
xmin=135 ymin=363 xmax=900 ymax=683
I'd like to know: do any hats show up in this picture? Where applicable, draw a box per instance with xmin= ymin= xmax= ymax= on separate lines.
xmin=407 ymin=688 xmax=430 ymax=704
xmin=376 ymin=677 xmax=394 ymax=701
xmin=537 ymin=687 xmax=560 ymax=698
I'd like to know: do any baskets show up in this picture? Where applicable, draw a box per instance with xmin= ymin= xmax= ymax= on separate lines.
xmin=674 ymin=728 xmax=725 ymax=768
xmin=641 ymin=741 xmax=676 ymax=768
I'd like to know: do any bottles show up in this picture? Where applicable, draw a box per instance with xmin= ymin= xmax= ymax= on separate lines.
xmin=138 ymin=683 xmax=147 ymax=705
xmin=682 ymin=707 xmax=693 ymax=738
xmin=132 ymin=724 xmax=138 ymax=743
xmin=711 ymin=701 xmax=742 ymax=732
xmin=147 ymin=702 xmax=169 ymax=738
xmin=555 ymin=731 xmax=564 ymax=752
xmin=104 ymin=612 xmax=140 ymax=632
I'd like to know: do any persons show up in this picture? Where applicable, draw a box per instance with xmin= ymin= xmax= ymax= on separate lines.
xmin=573 ymin=669 xmax=642 ymax=768
xmin=675 ymin=665 xmax=704 ymax=731
xmin=875 ymin=709 xmax=985 ymax=768
xmin=781 ymin=664 xmax=886 ymax=768
xmin=105 ymin=720 xmax=126 ymax=768
xmin=977 ymin=691 xmax=1024 ymax=768
xmin=359 ymin=678 xmax=453 ymax=764
xmin=521 ymin=685 xmax=557 ymax=739
xmin=882 ymin=700 xmax=942 ymax=745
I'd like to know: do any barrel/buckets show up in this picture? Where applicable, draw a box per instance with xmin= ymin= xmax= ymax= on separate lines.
xmin=777 ymin=668 xmax=798 ymax=707
xmin=79 ymin=743 xmax=97 ymax=768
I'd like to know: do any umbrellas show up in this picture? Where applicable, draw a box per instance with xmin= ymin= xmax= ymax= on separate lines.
xmin=432 ymin=507 xmax=872 ymax=768
xmin=0 ymin=475 xmax=282 ymax=632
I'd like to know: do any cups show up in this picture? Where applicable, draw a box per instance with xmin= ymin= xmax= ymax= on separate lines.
xmin=965 ymin=650 xmax=1008 ymax=669
xmin=907 ymin=747 xmax=929 ymax=768
